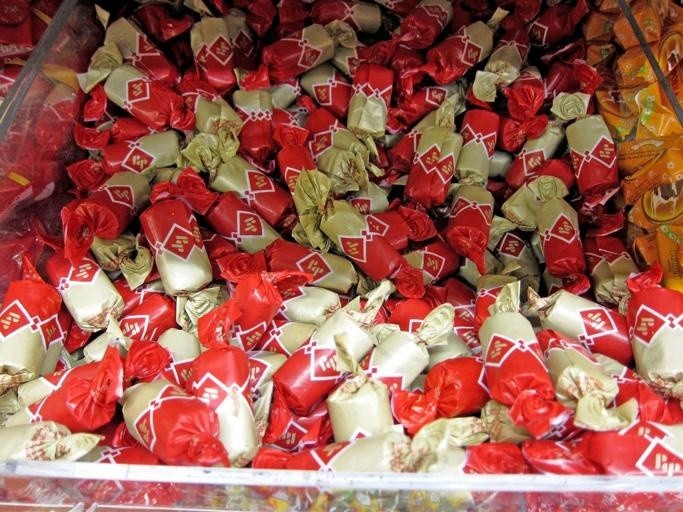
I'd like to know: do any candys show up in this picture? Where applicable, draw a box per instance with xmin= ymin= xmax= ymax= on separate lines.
xmin=0 ymin=0 xmax=683 ymax=512
